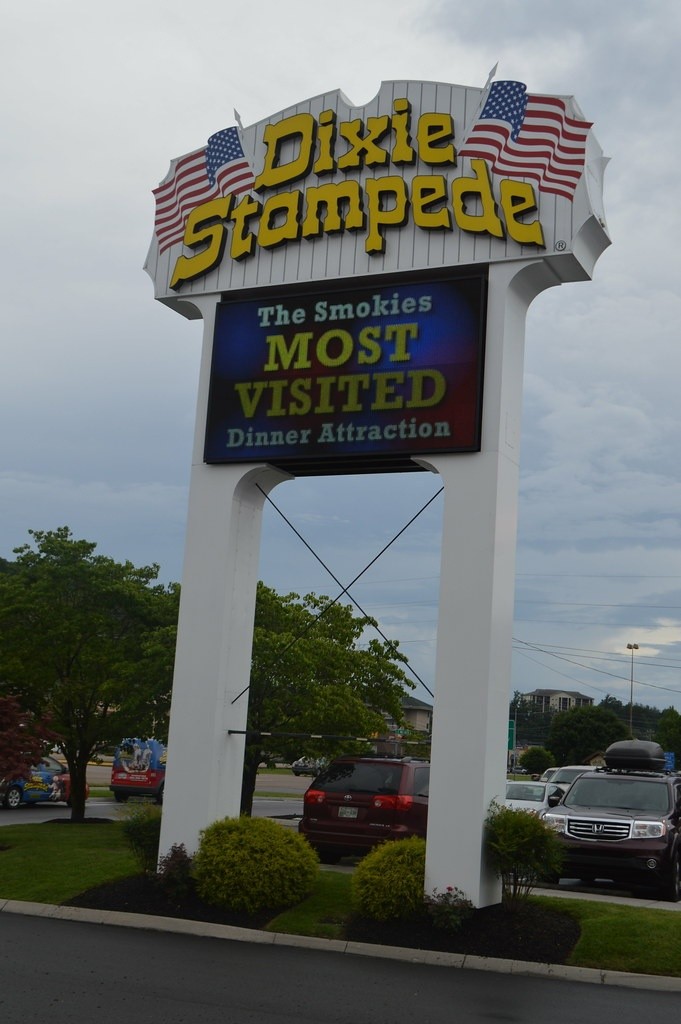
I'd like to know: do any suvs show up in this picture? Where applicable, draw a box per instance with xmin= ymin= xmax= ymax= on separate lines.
xmin=544 ymin=740 xmax=681 ymax=901
xmin=108 ymin=737 xmax=167 ymax=804
xmin=298 ymin=757 xmax=430 ymax=863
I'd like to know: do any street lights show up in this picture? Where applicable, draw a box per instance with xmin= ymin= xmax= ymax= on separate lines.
xmin=626 ymin=643 xmax=639 ymax=739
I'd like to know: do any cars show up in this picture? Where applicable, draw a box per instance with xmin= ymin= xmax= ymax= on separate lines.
xmin=292 ymin=755 xmax=328 ymax=776
xmin=0 ymin=755 xmax=89 ymax=810
xmin=532 ymin=768 xmax=559 ymax=792
xmin=506 ymin=781 xmax=566 ymax=830
xmin=512 ymin=766 xmax=528 ymax=775
xmin=540 ymin=765 xmax=606 ymax=802
xmin=51 ymin=744 xmax=62 ymax=754
